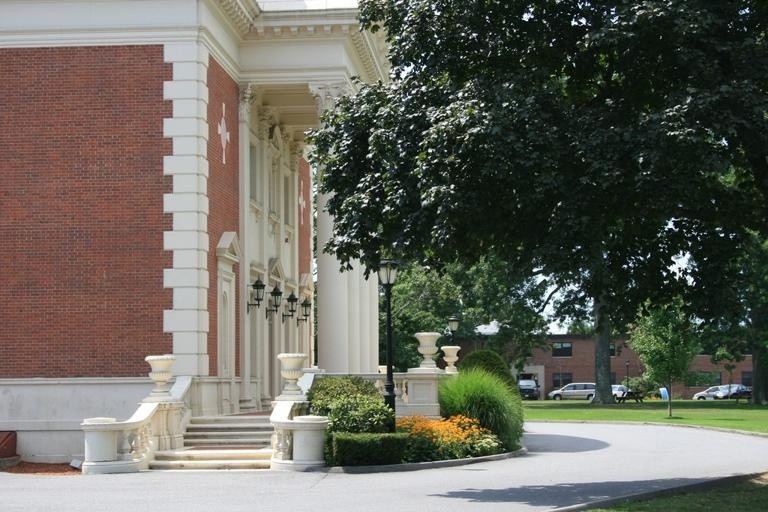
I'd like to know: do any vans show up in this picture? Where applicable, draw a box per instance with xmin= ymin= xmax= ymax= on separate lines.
xmin=548 ymin=382 xmax=596 ymax=400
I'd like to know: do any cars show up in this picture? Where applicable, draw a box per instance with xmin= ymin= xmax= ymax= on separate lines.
xmin=690 ymin=385 xmax=726 ymax=401
xmin=713 ymin=383 xmax=747 ymax=399
xmin=610 ymin=384 xmax=632 ymax=401
xmin=514 ymin=378 xmax=542 ymax=400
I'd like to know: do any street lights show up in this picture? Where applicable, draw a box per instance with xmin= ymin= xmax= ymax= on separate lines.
xmin=625 ymin=359 xmax=630 ymax=393
xmin=375 ymin=253 xmax=399 ymax=434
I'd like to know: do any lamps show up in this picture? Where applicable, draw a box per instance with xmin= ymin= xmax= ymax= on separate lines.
xmin=246 ymin=274 xmax=311 ymax=326
